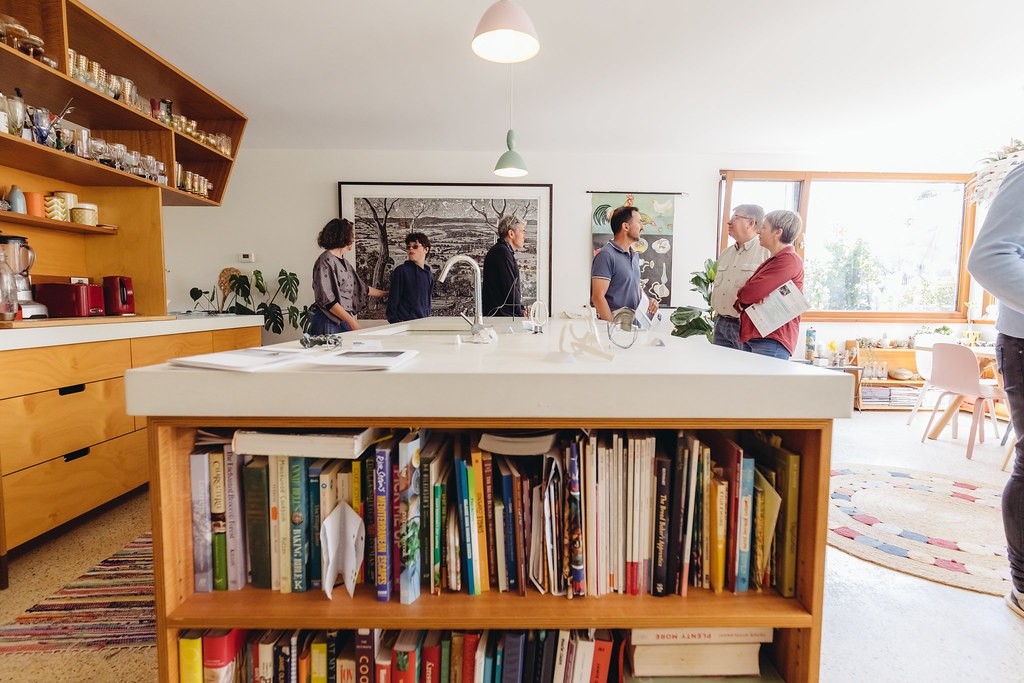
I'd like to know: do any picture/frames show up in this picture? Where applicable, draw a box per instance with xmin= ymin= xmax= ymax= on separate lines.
xmin=338 ymin=181 xmax=553 ymax=328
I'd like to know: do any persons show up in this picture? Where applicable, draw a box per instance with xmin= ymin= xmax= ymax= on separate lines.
xmin=386 ymin=233 xmax=436 ymax=324
xmin=589 ymin=206 xmax=660 ymax=330
xmin=781 ymin=287 xmax=787 ymax=295
xmin=308 ymin=218 xmax=390 ymax=336
xmin=734 ymin=209 xmax=804 ymax=360
xmin=482 ymin=215 xmax=530 ymax=317
xmin=965 ymin=164 xmax=1024 ymax=619
xmin=709 ymin=203 xmax=771 ymax=353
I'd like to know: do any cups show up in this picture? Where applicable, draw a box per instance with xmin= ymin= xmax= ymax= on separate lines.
xmin=859 ymin=360 xmax=888 ymax=380
xmin=1 ymin=47 xmax=232 ymax=199
xmin=23 ymin=190 xmax=98 ymax=227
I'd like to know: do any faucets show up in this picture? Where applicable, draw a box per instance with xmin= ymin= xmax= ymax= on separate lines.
xmin=437 ymin=255 xmax=484 ymax=334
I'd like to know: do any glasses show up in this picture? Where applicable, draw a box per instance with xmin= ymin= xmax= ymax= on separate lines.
xmin=405 ymin=244 xmax=424 ymax=250
xmin=343 ymin=218 xmax=354 ymax=240
xmin=729 ymin=214 xmax=758 ymax=226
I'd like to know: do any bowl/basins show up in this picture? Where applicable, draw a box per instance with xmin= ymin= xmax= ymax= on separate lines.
xmin=961 ymin=331 xmax=981 ymax=346
xmin=889 ymin=367 xmax=913 ymax=380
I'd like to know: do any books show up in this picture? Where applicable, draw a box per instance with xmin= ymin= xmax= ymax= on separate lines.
xmin=186 ymin=424 xmax=802 ymax=683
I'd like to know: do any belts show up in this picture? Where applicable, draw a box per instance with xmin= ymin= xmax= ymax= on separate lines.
xmin=718 ymin=315 xmax=739 ymax=322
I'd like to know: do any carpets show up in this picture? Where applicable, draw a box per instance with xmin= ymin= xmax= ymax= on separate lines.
xmin=827 ymin=466 xmax=1014 ymax=596
xmin=1 ymin=526 xmax=158 ymax=657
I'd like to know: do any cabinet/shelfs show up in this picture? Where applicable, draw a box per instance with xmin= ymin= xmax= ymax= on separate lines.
xmin=0 ymin=0 xmax=266 ymax=591
xmin=846 ymin=339 xmax=946 ymax=411
xmin=145 ymin=415 xmax=833 ymax=683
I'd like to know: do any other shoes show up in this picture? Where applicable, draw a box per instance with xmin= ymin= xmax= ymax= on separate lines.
xmin=1005 ymin=585 xmax=1024 ymax=618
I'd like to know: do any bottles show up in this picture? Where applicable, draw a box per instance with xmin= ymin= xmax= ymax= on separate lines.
xmin=8 ymin=185 xmax=27 ymax=215
xmin=0 ymin=252 xmax=18 ymax=321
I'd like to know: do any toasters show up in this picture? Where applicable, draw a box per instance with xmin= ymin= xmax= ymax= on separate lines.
xmin=31 ymin=282 xmax=105 ymax=317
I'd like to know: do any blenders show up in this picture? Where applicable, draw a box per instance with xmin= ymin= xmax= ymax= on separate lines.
xmin=0 ymin=235 xmax=49 ymax=319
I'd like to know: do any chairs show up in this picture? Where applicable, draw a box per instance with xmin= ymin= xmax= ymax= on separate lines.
xmin=906 ymin=334 xmax=1005 ymax=459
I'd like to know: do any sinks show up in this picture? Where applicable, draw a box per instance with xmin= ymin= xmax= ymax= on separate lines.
xmin=389 ymin=330 xmax=474 ymax=336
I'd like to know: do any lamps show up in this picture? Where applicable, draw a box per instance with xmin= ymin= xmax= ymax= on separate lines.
xmin=472 ymin=0 xmax=541 ymax=63
xmin=494 ymin=62 xmax=528 ymax=178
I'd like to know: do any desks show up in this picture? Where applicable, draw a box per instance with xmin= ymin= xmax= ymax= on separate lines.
xmin=915 ymin=345 xmax=1014 ymax=447
xmin=825 ymin=365 xmax=866 ymax=412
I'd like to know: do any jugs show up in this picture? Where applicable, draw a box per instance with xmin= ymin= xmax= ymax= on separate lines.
xmin=105 ymin=275 xmax=134 ymax=314
